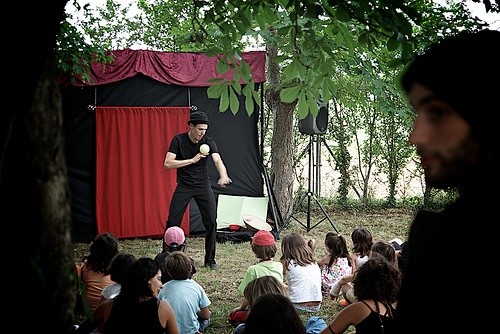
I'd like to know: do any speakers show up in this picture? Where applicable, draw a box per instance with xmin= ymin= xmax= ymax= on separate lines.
xmin=298 ymin=89 xmax=331 ymax=135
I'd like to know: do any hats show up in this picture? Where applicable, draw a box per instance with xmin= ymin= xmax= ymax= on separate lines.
xmin=251 ymin=230 xmax=274 ymax=246
xmin=187 ymin=111 xmax=211 ymax=125
xmin=165 ymin=226 xmax=186 ymax=247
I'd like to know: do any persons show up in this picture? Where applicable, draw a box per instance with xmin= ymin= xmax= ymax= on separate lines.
xmin=157 ymin=250 xmax=211 ymax=334
xmin=228 ymin=230 xmax=284 ymax=323
xmin=280 ymin=233 xmax=322 ymax=313
xmin=233 ymin=274 xmax=306 ymax=334
xmin=76 ymin=258 xmax=179 ymax=334
xmin=154 ymin=226 xmax=197 ymax=279
xmin=163 ymin=111 xmax=232 ymax=270
xmin=317 ymin=233 xmax=356 ymax=296
xmin=81 ymin=233 xmax=118 ymax=316
xmin=351 ymin=229 xmax=373 ymax=272
xmin=101 ymin=253 xmax=137 ymax=302
xmin=319 ymin=242 xmax=401 ymax=334
xmin=393 ymin=30 xmax=500 ymax=334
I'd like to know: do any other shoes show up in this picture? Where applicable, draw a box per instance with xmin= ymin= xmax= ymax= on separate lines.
xmin=204 ymin=262 xmax=217 ymax=270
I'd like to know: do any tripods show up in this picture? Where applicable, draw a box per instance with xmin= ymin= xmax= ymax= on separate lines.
xmin=276 ymin=134 xmax=342 ymax=238
